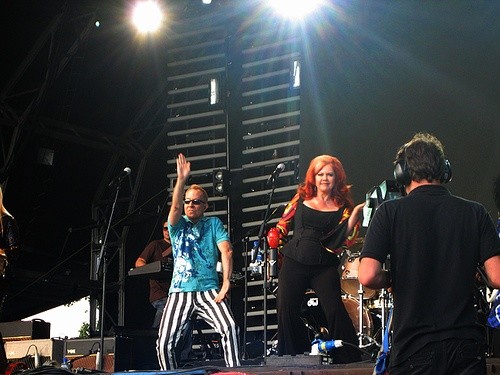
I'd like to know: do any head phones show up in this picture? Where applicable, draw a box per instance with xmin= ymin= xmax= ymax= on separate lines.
xmin=393 ymin=143 xmax=454 ymax=186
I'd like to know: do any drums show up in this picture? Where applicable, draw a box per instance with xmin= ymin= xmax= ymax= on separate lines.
xmin=298 ymin=292 xmax=331 ymax=354
xmin=342 ymin=296 xmax=374 ymax=344
xmin=341 ymin=254 xmax=377 ymax=300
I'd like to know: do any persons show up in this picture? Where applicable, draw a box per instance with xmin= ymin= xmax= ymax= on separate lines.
xmin=156 ymin=153 xmax=242 ymax=371
xmin=358 ymin=132 xmax=500 ymax=375
xmin=135 ymin=221 xmax=174 ymax=327
xmin=267 ymin=155 xmax=366 ymax=364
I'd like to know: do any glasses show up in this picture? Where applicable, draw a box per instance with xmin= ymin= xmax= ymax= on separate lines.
xmin=163 ymin=227 xmax=169 ymax=231
xmin=184 ymin=199 xmax=206 ymax=205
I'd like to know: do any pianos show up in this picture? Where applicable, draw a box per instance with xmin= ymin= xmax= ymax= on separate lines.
xmin=128 ymin=259 xmax=242 ymax=362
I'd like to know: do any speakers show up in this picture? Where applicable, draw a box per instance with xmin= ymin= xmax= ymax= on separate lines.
xmin=63 ymin=336 xmax=159 ymax=373
xmin=4 ymin=338 xmax=63 ymax=368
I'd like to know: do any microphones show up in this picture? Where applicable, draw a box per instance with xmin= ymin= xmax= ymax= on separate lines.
xmin=266 ymin=163 xmax=286 ymax=186
xmin=95 ymin=347 xmax=101 ymax=371
xmin=35 ymin=348 xmax=41 ymax=368
xmin=108 ymin=167 xmax=132 ymax=187
